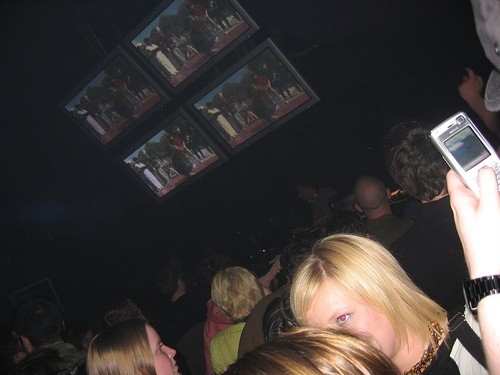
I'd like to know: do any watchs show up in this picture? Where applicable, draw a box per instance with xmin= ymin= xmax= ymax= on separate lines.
xmin=462 ymin=275 xmax=500 ymax=311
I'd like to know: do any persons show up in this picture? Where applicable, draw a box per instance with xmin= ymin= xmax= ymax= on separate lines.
xmin=459 ymin=66 xmax=500 ymax=137
xmin=133 ymin=0 xmax=245 ymax=79
xmin=197 ymin=61 xmax=303 ymax=145
xmin=235 ymin=213 xmax=371 ymax=357
xmin=0 ymin=296 xmax=145 ymax=375
xmin=446 ymin=167 xmax=500 ymax=375
xmin=133 ymin=263 xmax=206 ymax=344
xmin=125 ymin=124 xmax=217 ymax=197
xmin=380 ymin=116 xmax=476 ymax=310
xmin=86 ymin=318 xmax=180 ymax=375
xmin=296 ymin=179 xmax=332 ymax=221
xmin=203 ymin=255 xmax=273 ymax=375
xmin=73 ymin=55 xmax=156 ymax=141
xmin=208 ymin=266 xmax=268 ymax=375
xmin=289 ymin=234 xmax=487 ymax=375
xmin=351 ymin=172 xmax=416 ymax=250
xmin=220 ymin=324 xmax=402 ymax=375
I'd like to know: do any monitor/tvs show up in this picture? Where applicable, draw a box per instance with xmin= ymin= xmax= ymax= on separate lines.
xmin=185 ymin=35 xmax=321 ymax=157
xmin=58 ymin=44 xmax=171 ymax=152
xmin=121 ymin=0 xmax=261 ymax=96
xmin=115 ymin=105 xmax=230 ymax=205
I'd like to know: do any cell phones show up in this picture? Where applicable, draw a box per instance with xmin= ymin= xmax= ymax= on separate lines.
xmin=428 ymin=110 xmax=500 ymax=199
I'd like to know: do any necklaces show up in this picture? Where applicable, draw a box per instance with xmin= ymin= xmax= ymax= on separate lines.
xmin=404 ymin=321 xmax=444 ymax=375
